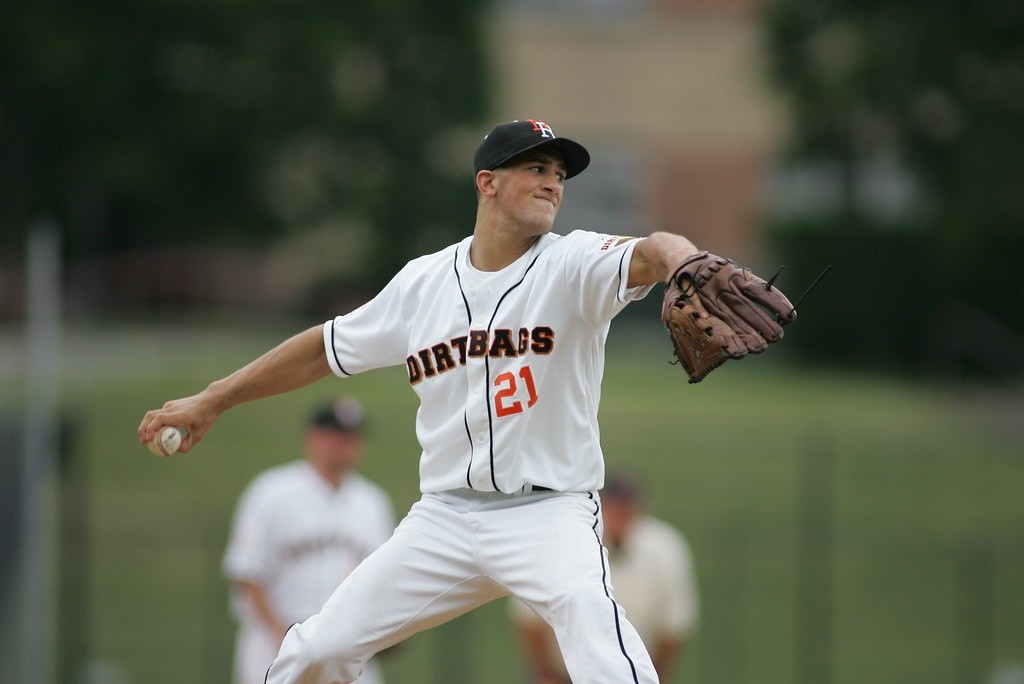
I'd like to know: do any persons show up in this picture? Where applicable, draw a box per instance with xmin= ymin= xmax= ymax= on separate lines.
xmin=516 ymin=479 xmax=701 ymax=684
xmin=136 ymin=120 xmax=796 ymax=684
xmin=221 ymin=399 xmax=396 ymax=684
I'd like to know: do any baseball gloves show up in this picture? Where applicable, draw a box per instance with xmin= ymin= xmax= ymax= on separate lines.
xmin=661 ymin=250 xmax=798 ymax=385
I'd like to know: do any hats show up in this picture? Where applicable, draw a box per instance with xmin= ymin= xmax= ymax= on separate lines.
xmin=311 ymin=401 xmax=365 ymax=432
xmin=474 ymin=119 xmax=591 ymax=180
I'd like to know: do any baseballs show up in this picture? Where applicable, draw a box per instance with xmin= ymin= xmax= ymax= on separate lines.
xmin=146 ymin=428 xmax=180 ymax=459
